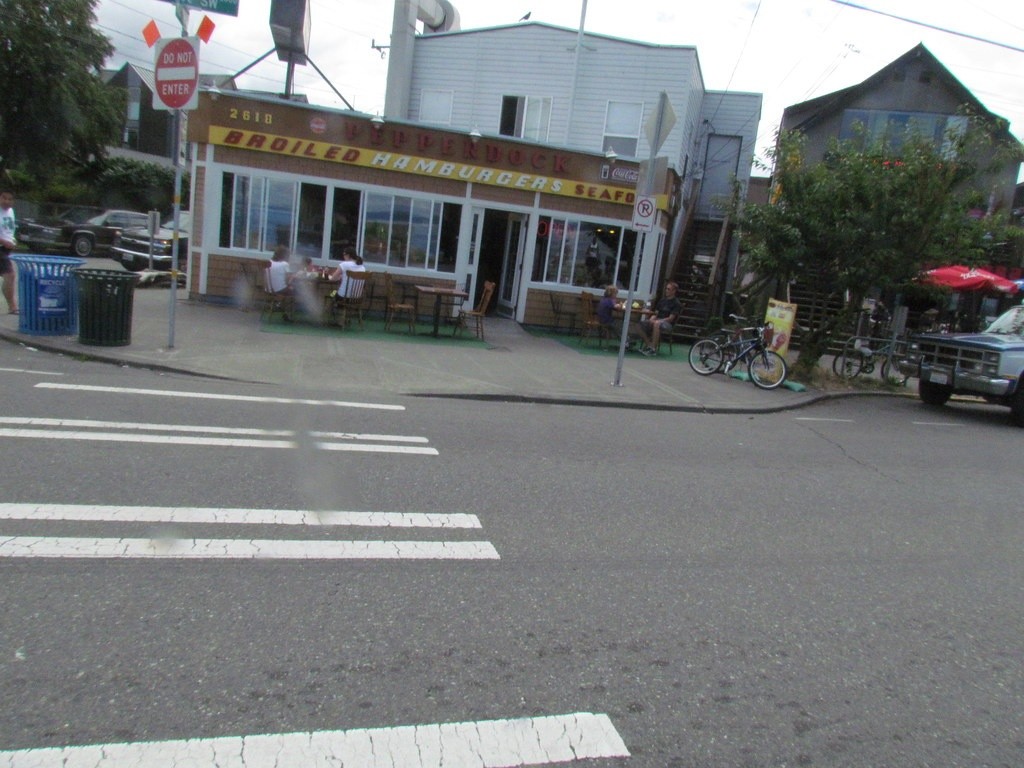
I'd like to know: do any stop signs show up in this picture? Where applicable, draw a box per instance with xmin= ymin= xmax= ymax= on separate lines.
xmin=155 ymin=39 xmax=200 ymax=109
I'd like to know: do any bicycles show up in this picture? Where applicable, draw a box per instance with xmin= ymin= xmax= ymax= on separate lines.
xmin=833 ymin=337 xmax=912 ymax=384
xmin=688 ymin=313 xmax=788 ymax=390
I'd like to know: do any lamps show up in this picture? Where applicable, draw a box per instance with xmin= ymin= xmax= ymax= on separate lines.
xmin=468 ymin=123 xmax=482 ymax=144
xmin=606 ymin=146 xmax=618 ymax=163
xmin=207 ymin=80 xmax=222 ymax=101
xmin=370 ymin=110 xmax=384 ymax=130
xmin=371 ymin=39 xmax=390 ymax=60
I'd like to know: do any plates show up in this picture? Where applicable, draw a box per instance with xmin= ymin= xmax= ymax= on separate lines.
xmin=632 ymin=307 xmax=642 ymax=309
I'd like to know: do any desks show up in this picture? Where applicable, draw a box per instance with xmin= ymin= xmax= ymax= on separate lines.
xmin=135 ymin=272 xmax=185 ymax=288
xmin=293 ymin=277 xmax=340 ymax=325
xmin=613 ymin=307 xmax=655 ymax=353
xmin=415 ymin=286 xmax=468 ymax=338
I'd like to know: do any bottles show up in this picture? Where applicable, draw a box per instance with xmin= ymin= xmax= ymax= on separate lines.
xmin=321 ymin=270 xmax=325 ymax=279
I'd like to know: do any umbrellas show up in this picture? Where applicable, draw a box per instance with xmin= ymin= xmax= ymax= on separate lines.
xmin=912 ymin=264 xmax=1018 ymax=332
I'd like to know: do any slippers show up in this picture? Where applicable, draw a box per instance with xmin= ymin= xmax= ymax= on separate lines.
xmin=9 ymin=310 xmax=20 ymax=315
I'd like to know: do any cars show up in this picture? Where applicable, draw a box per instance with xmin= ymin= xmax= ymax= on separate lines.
xmin=900 ymin=305 xmax=1024 ymax=428
xmin=13 ymin=203 xmax=150 ymax=258
xmin=113 ymin=210 xmax=190 ymax=273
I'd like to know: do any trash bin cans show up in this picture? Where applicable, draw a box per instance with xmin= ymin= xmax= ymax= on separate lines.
xmin=10 ymin=254 xmax=86 ymax=335
xmin=72 ymin=268 xmax=139 ymax=347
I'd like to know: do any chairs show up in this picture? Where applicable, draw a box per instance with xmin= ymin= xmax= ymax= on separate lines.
xmin=384 ymin=271 xmax=495 ymax=342
xmin=240 ymin=260 xmax=377 ymax=332
xmin=577 ymin=291 xmax=686 ymax=356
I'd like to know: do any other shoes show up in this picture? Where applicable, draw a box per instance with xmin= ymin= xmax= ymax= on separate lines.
xmin=639 ymin=346 xmax=649 ymax=352
xmin=625 ymin=341 xmax=636 ymax=351
xmin=282 ymin=313 xmax=292 ymax=323
xmin=642 ymin=348 xmax=656 ymax=356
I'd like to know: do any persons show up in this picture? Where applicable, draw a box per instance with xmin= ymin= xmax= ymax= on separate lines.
xmin=331 ymin=247 xmax=366 ymax=327
xmin=591 ymin=268 xmax=603 ymax=288
xmin=264 ymin=246 xmax=308 ymax=322
xmin=0 ymin=186 xmax=19 ymax=315
xmin=586 ymin=237 xmax=599 ymax=258
xmin=636 ymin=282 xmax=681 ymax=356
xmin=597 ymin=285 xmax=637 ymax=351
xmin=869 ymin=301 xmax=892 ymax=351
